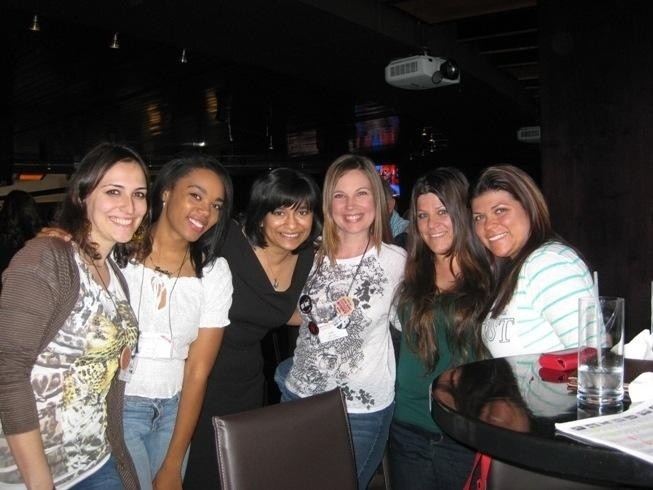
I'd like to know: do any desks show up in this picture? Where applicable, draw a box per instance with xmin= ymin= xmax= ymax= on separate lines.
xmin=430 ymin=347 xmax=653 ymax=490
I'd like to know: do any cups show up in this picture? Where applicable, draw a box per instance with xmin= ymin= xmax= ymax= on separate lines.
xmin=576 ymin=295 xmax=626 ymax=404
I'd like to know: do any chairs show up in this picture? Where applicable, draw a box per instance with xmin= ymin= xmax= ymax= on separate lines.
xmin=212 ymin=389 xmax=362 ymax=490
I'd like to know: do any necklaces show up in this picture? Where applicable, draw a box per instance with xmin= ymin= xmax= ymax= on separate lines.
xmin=265 ymin=249 xmax=285 ymax=289
xmin=89 ymin=255 xmax=127 ymax=331
xmin=147 ymin=253 xmax=183 ymax=277
xmin=335 ymin=233 xmax=370 ymax=316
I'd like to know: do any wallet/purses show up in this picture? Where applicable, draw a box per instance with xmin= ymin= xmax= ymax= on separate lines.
xmin=538 ymin=346 xmax=597 ymax=372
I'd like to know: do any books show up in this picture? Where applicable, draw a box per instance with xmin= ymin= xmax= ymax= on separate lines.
xmin=555 ymin=398 xmax=653 ymax=463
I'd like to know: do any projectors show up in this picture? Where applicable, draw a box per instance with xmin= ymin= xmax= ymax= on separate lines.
xmin=385 ymin=55 xmax=461 ymax=91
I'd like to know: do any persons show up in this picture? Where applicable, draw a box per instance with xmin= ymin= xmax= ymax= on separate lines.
xmin=385 ymin=165 xmax=495 ymax=489
xmin=378 ymin=178 xmax=410 ymax=238
xmin=0 ymin=191 xmax=51 ymax=283
xmin=182 ymin=166 xmax=315 ymax=490
xmin=279 ymin=155 xmax=412 ymax=490
xmin=467 ymin=164 xmax=607 ymax=358
xmin=36 ymin=154 xmax=233 ymax=490
xmin=0 ymin=140 xmax=154 ymax=490
xmin=479 ymin=353 xmax=578 ymax=432
